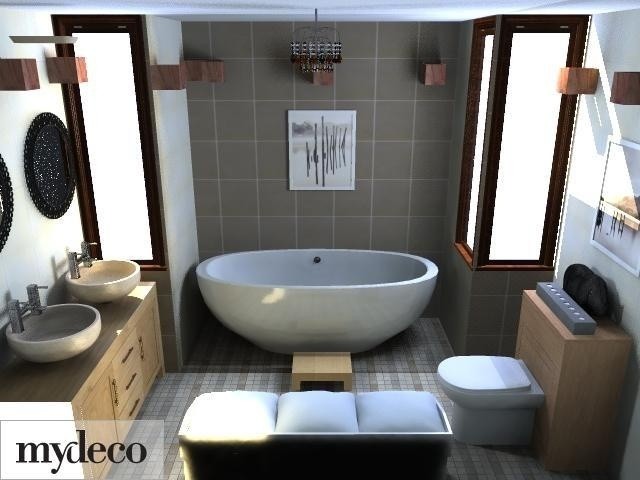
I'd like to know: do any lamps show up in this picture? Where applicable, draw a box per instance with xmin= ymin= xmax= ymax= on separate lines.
xmin=286 ymin=8 xmax=343 ymax=73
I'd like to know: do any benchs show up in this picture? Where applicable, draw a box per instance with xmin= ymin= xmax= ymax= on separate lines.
xmin=175 ymin=390 xmax=455 ymax=480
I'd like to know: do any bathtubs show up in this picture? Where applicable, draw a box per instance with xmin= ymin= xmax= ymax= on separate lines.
xmin=194 ymin=247 xmax=440 ymax=360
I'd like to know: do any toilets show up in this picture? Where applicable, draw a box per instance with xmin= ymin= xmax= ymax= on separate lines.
xmin=436 ymin=356 xmax=545 ymax=446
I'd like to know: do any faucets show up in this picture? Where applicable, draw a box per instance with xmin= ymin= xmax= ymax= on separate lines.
xmin=8 ymin=285 xmax=48 ymax=332
xmin=67 ymin=241 xmax=97 ymax=279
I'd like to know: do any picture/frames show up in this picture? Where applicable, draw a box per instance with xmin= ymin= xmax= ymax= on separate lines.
xmin=589 ymin=134 xmax=640 ymax=280
xmin=287 ymin=110 xmax=356 ymax=192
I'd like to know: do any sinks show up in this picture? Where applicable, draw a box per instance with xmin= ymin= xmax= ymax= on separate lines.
xmin=63 ymin=259 xmax=141 ymax=303
xmin=6 ymin=304 xmax=101 ymax=364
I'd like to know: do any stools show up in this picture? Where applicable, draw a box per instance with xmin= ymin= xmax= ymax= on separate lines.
xmin=289 ymin=350 xmax=354 ymax=391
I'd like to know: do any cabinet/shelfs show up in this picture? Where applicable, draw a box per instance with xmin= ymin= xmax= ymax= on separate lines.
xmin=0 ymin=280 xmax=168 ymax=479
xmin=514 ymin=289 xmax=633 ymax=477
